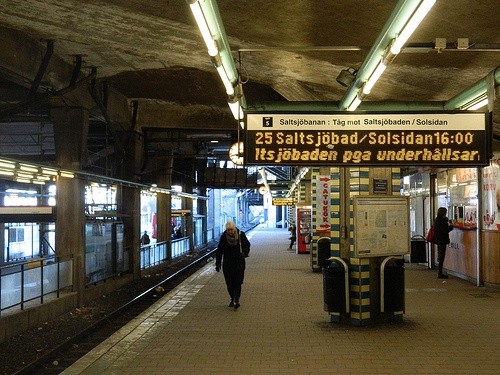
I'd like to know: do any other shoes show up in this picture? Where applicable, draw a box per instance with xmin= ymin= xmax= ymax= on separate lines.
xmin=235 ymin=296 xmax=240 ymax=309
xmin=229 ymin=296 xmax=234 ymax=307
xmin=437 ymin=273 xmax=448 ymax=278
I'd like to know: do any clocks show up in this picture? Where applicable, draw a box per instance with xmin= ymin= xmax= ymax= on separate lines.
xmin=229 ymin=141 xmax=244 ymax=166
xmin=259 ymin=186 xmax=268 ymax=195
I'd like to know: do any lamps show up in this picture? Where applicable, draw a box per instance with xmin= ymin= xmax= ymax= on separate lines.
xmin=258 ymin=166 xmax=310 ymax=198
xmin=185 ymin=0 xmax=222 ymax=59
xmin=52 ymin=176 xmax=56 ymax=182
xmin=16 ymin=177 xmax=30 ymax=183
xmin=41 ymin=167 xmax=58 ymax=175
xmin=385 ymin=0 xmax=437 ymax=63
xmin=36 ymin=174 xmax=50 ymax=180
xmin=460 ymin=93 xmax=489 ymax=110
xmin=360 ymin=58 xmax=390 ymax=98
xmin=19 ymin=162 xmax=39 ymax=172
xmin=33 ymin=179 xmax=46 ymax=185
xmin=226 ymin=83 xmax=249 ymax=130
xmin=0 ymin=168 xmax=15 ymax=176
xmin=171 ymin=185 xmax=182 ymax=192
xmin=0 ymin=159 xmax=17 ymax=169
xmin=17 ymin=171 xmax=33 ymax=178
xmin=149 ymin=188 xmax=171 ymax=194
xmin=60 ymin=171 xmax=75 ymax=179
xmin=345 ymin=92 xmax=364 ymax=112
xmin=213 ymin=60 xmax=236 ymax=100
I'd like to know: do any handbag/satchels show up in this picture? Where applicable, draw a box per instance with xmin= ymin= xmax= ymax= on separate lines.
xmin=427 ymin=225 xmax=436 ymax=244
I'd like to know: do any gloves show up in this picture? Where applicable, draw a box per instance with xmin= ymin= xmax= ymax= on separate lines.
xmin=216 ymin=263 xmax=220 ymax=272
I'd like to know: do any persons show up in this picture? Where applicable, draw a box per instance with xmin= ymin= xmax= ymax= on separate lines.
xmin=466 ymin=211 xmax=476 ymax=221
xmin=140 ymin=231 xmax=150 ymax=250
xmin=171 ymin=223 xmax=182 ymax=240
xmin=434 ymin=207 xmax=453 ymax=279
xmin=494 ymin=187 xmax=500 ymax=224
xmin=216 ymin=221 xmax=251 ymax=309
xmin=287 ymin=227 xmax=296 ymax=250
